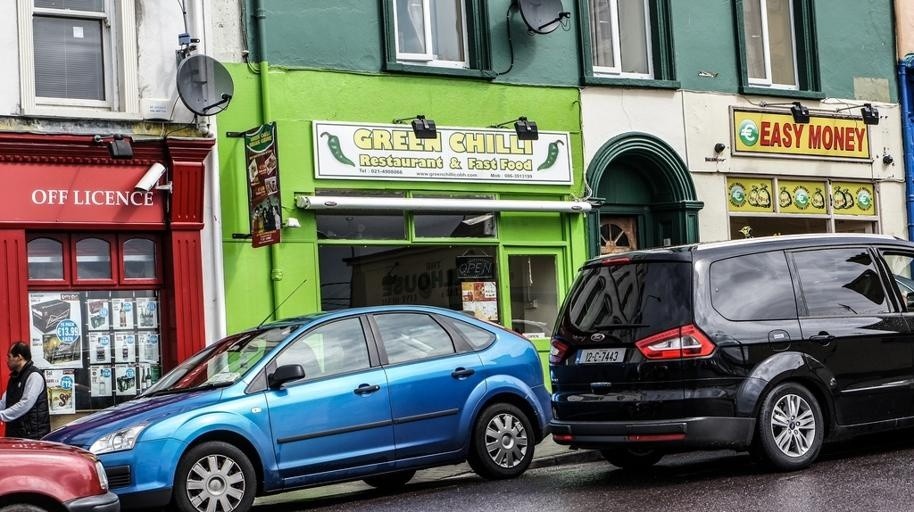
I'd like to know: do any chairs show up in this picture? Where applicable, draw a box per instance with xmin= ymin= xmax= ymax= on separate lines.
xmin=335 ymin=323 xmax=366 ymax=369
xmin=281 ymin=335 xmax=320 ymax=382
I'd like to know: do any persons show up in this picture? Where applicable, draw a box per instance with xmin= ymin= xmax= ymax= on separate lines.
xmin=251 ymin=198 xmax=281 ymax=233
xmin=0 ymin=342 xmax=51 ymax=441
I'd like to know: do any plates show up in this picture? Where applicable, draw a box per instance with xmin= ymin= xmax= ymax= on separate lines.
xmin=248 ymin=158 xmax=259 ymax=183
xmin=264 ymin=153 xmax=277 ymax=175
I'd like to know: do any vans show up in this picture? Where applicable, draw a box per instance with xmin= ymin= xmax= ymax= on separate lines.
xmin=545 ymin=234 xmax=914 ymax=477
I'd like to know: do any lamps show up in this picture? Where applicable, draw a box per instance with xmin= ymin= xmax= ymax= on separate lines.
xmin=757 ymin=102 xmax=813 ymax=123
xmin=391 ymin=111 xmax=441 ymax=141
xmin=89 ymin=131 xmax=137 ymax=159
xmin=483 ymin=115 xmax=539 ymax=140
xmin=832 ymin=103 xmax=880 ymax=126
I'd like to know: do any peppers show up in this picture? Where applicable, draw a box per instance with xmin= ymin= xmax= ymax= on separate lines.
xmin=537 ymin=140 xmax=564 ymax=170
xmin=321 ymin=132 xmax=355 ymax=167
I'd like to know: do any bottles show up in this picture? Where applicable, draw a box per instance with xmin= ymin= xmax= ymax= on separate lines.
xmin=145 ymin=334 xmax=157 ymax=360
xmin=96 ymin=337 xmax=105 ymax=362
xmin=121 ymin=336 xmax=128 ymax=361
xmin=91 ymin=368 xmax=105 ymax=395
xmin=140 ymin=367 xmax=147 ymax=392
xmin=146 ymin=368 xmax=153 ymax=389
xmin=139 ymin=307 xmax=154 ymax=328
xmin=119 ymin=303 xmax=128 ymax=328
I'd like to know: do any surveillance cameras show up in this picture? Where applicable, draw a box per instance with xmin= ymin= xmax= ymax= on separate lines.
xmin=133 ymin=163 xmax=167 ymax=192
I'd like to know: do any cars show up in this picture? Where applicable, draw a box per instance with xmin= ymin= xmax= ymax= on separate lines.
xmin=39 ymin=278 xmax=554 ymax=511
xmin=41 ymin=376 xmax=109 ymax=410
xmin=0 ymin=437 xmax=120 ymax=512
